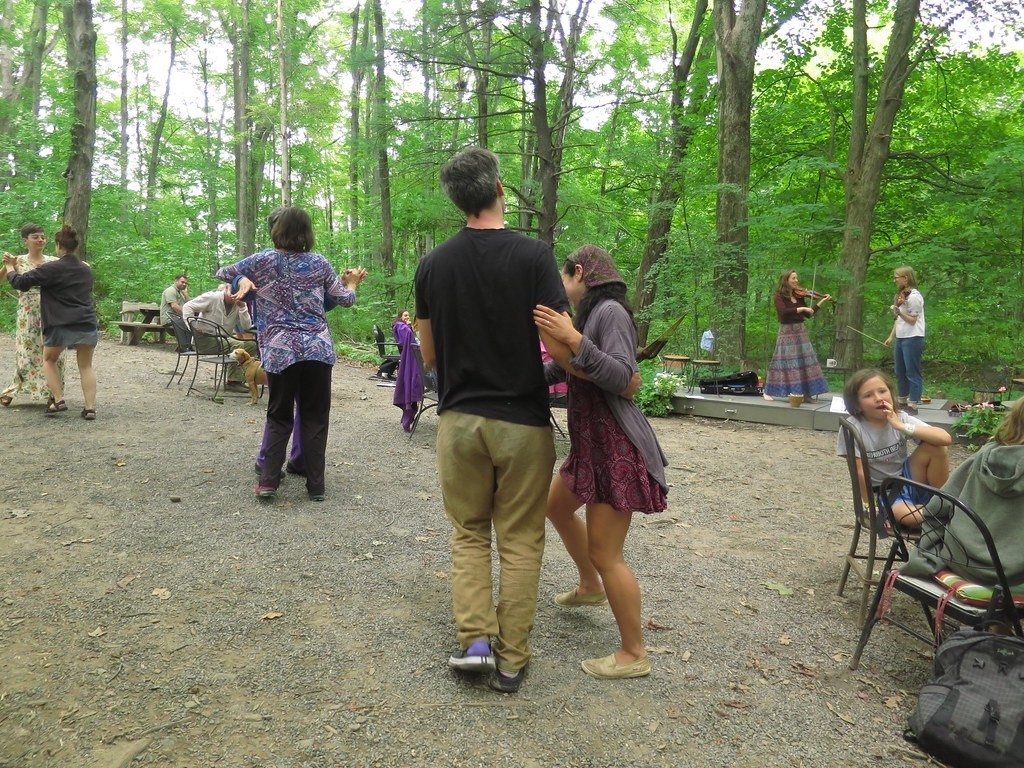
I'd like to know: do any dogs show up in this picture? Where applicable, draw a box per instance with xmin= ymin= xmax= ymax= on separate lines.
xmin=227 ymin=348 xmax=267 ymax=406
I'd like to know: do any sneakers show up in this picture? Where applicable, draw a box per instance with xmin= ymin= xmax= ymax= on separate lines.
xmin=447 ymin=641 xmax=495 ymax=673
xmin=491 ymin=660 xmax=524 ymax=693
xmin=554 ymin=586 xmax=609 ymax=607
xmin=580 ymin=651 xmax=652 ymax=679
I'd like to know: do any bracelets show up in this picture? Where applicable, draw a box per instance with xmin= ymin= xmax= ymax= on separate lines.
xmin=902 ymin=423 xmax=915 ymax=439
xmin=864 ymin=500 xmax=879 ymax=513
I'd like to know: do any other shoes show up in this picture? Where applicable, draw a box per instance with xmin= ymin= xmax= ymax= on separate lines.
xmin=763 ymin=391 xmax=773 ymax=401
xmin=255 ymin=484 xmax=277 ymax=497
xmin=897 ymin=401 xmax=918 ymax=416
xmin=226 ymin=380 xmax=249 ymax=393
xmin=802 ymin=395 xmax=818 ymax=404
xmin=0 ymin=395 xmax=13 ymax=407
xmin=255 ymin=462 xmax=286 ymax=478
xmin=286 ymin=461 xmax=306 ymax=476
xmin=308 ymin=492 xmax=326 ymax=501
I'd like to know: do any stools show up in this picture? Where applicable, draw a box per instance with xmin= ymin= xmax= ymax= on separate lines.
xmin=688 ymin=359 xmax=721 ymax=396
xmin=664 ymin=355 xmax=689 ymax=391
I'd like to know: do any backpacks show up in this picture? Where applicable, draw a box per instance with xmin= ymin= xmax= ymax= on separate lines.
xmin=906 ymin=620 xmax=1024 ymax=768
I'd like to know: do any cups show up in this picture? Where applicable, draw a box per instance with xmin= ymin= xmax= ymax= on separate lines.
xmin=788 ymin=394 xmax=804 ymax=408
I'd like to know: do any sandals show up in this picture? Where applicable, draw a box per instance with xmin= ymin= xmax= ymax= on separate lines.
xmin=44 ymin=399 xmax=69 ymax=412
xmin=81 ymin=408 xmax=97 ymax=419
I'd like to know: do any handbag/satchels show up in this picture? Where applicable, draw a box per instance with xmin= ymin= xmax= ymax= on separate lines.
xmin=377 ymin=360 xmax=399 ymax=381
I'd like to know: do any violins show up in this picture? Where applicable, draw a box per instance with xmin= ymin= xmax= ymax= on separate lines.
xmin=792 ymin=285 xmax=837 ymax=305
xmin=893 ymin=286 xmax=911 ymax=321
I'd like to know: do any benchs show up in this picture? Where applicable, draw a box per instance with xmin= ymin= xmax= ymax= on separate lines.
xmin=119 ymin=322 xmax=174 ymax=346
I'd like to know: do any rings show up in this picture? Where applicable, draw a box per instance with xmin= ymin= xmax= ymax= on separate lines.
xmin=812 ymin=310 xmax=813 ymax=311
xmin=546 ymin=321 xmax=549 ymax=326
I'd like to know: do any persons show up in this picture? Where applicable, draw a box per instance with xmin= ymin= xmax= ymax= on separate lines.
xmin=160 ymin=275 xmax=195 ymax=353
xmin=919 ymin=397 xmax=1024 ymax=637
xmin=0 ymin=223 xmax=98 ymax=421
xmin=415 ymin=147 xmax=643 ymax=692
xmin=391 ymin=310 xmax=420 ymax=345
xmin=215 ymin=207 xmax=368 ymax=501
xmin=836 ymin=368 xmax=952 ymax=529
xmin=885 ymin=266 xmax=925 ymax=417
xmin=532 ymin=244 xmax=669 ymax=682
xmin=182 ymin=283 xmax=259 ymax=394
xmin=763 ymin=270 xmax=832 ymax=404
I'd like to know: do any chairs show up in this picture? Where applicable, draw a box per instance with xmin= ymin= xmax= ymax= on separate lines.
xmin=839 ymin=417 xmax=1024 ymax=679
xmin=373 ymin=325 xmax=402 ymax=380
xmin=409 ymin=343 xmax=440 ymax=439
xmin=165 ymin=311 xmax=265 ymax=399
